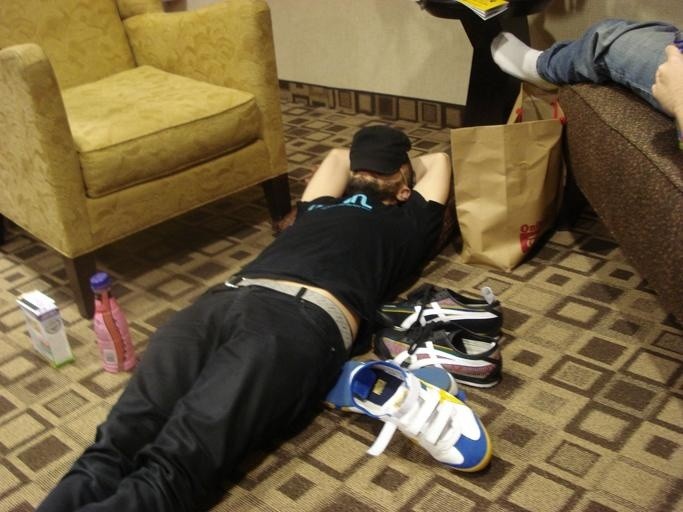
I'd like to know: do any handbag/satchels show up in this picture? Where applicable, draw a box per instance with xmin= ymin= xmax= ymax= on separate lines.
xmin=450 ymin=83 xmax=567 ymax=274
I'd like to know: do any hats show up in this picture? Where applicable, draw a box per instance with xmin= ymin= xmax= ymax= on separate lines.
xmin=350 ymin=126 xmax=411 ymax=175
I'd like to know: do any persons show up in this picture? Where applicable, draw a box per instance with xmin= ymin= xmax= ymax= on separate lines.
xmin=35 ymin=124 xmax=451 ymax=512
xmin=490 ymin=17 xmax=683 ymax=150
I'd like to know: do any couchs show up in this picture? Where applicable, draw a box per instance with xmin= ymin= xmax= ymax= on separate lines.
xmin=0 ymin=0 xmax=291 ymax=320
xmin=556 ymin=81 xmax=683 ymax=332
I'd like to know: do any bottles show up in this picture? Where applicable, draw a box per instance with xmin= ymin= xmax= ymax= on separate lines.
xmin=90 ymin=272 xmax=137 ymax=373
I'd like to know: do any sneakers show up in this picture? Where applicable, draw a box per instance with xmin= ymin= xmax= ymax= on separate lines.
xmin=321 ymin=360 xmax=465 ymax=416
xmin=375 ymin=282 xmax=503 ymax=341
xmin=348 ymin=362 xmax=492 ymax=473
xmin=375 ymin=320 xmax=502 ymax=389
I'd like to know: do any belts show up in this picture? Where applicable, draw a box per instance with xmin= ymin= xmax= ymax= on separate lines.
xmin=224 ymin=275 xmax=353 ymax=349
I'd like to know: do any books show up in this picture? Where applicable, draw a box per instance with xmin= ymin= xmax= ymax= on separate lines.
xmin=457 ymin=0 xmax=509 ymax=21
